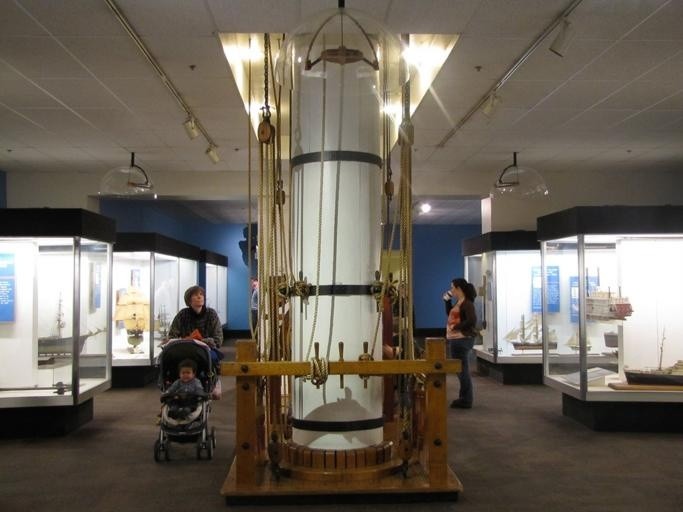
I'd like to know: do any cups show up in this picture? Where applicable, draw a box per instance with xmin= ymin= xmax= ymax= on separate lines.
xmin=444 ymin=291 xmax=453 ymax=301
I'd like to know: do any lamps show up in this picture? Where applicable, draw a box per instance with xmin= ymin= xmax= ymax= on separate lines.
xmin=273 ymin=0 xmax=410 ymax=94
xmin=99 ymin=151 xmax=157 ymax=201
xmin=490 ymin=153 xmax=549 ymax=198
xmin=549 ymin=15 xmax=577 ymax=59
xmin=481 ymin=91 xmax=502 ymax=118
xmin=182 ymin=111 xmax=220 ymax=166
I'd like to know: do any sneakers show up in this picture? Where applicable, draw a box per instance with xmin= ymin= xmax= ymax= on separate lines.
xmin=451 ymin=400 xmax=471 ymax=409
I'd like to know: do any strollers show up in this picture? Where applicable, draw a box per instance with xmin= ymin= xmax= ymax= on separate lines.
xmin=151 ymin=337 xmax=217 ymax=464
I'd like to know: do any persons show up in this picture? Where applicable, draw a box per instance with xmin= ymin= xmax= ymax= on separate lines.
xmin=248 ymin=276 xmax=259 ymax=343
xmin=153 ymin=362 xmax=204 ymax=427
xmin=442 ymin=277 xmax=477 ymax=409
xmin=158 ymin=285 xmax=224 ymax=349
